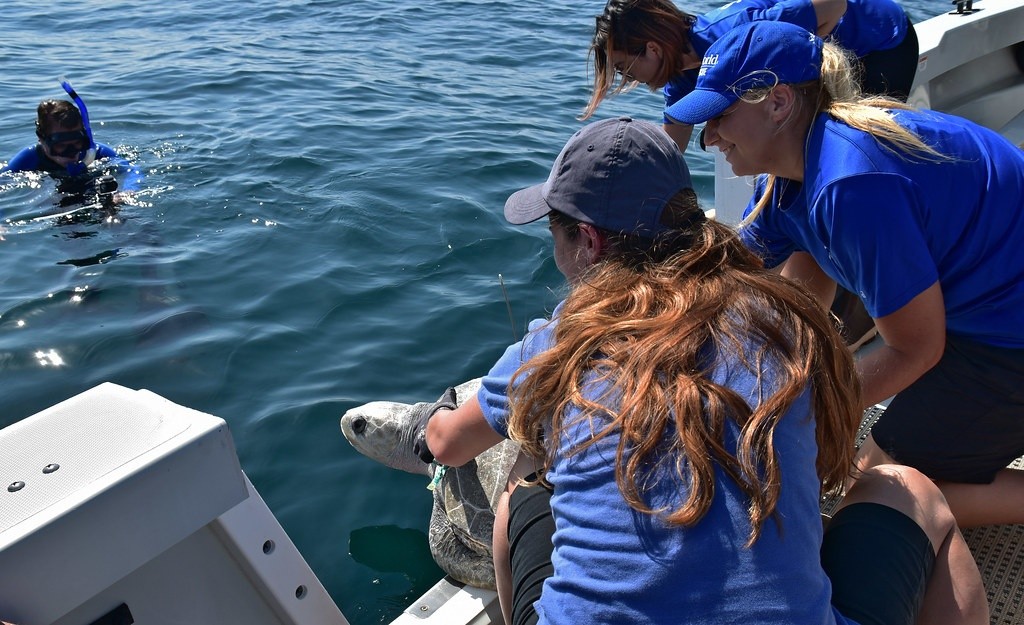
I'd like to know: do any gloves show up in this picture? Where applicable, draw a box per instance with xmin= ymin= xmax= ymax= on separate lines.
xmin=411 ymin=385 xmax=459 ymax=464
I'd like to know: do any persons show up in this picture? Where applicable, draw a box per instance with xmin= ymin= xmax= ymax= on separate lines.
xmin=412 ymin=115 xmax=990 ymax=625
xmin=665 ymin=20 xmax=1024 ymax=528
xmin=0 ymin=99 xmax=144 ymax=203
xmin=574 ymin=0 xmax=919 ymax=355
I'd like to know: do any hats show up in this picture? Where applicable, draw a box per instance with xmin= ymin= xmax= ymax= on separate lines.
xmin=664 ymin=20 xmax=823 ymax=126
xmin=503 ymin=116 xmax=694 ymax=238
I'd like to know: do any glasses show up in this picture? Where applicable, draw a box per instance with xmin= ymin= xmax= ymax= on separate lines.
xmin=617 ymin=50 xmax=644 ymax=78
xmin=45 ymin=130 xmax=89 ymax=157
